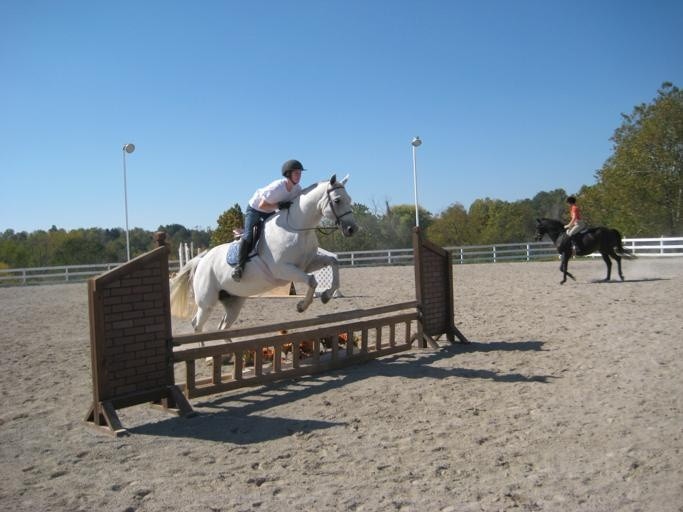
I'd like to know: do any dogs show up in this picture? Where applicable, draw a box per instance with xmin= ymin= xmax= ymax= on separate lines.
xmin=534 ymin=217 xmax=639 ymax=286
xmin=169 ymin=172 xmax=359 ymax=367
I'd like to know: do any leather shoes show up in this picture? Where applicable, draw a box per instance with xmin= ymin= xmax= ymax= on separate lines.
xmin=278 ymin=200 xmax=294 ymax=210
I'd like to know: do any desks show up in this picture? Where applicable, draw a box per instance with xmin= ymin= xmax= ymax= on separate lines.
xmin=122 ymin=142 xmax=136 ymax=261
xmin=410 ymin=136 xmax=425 ymax=226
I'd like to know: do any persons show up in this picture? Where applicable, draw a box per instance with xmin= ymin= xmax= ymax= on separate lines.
xmin=232 ymin=159 xmax=306 ymax=284
xmin=563 ymin=196 xmax=587 ymax=254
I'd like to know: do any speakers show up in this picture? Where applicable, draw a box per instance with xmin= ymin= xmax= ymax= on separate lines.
xmin=569 ymin=234 xmax=582 ymax=256
xmin=231 ymin=236 xmax=252 ymax=284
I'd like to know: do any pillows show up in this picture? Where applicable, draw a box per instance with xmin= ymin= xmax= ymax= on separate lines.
xmin=566 ymin=196 xmax=577 ymax=203
xmin=281 ymin=159 xmax=308 ymax=176
